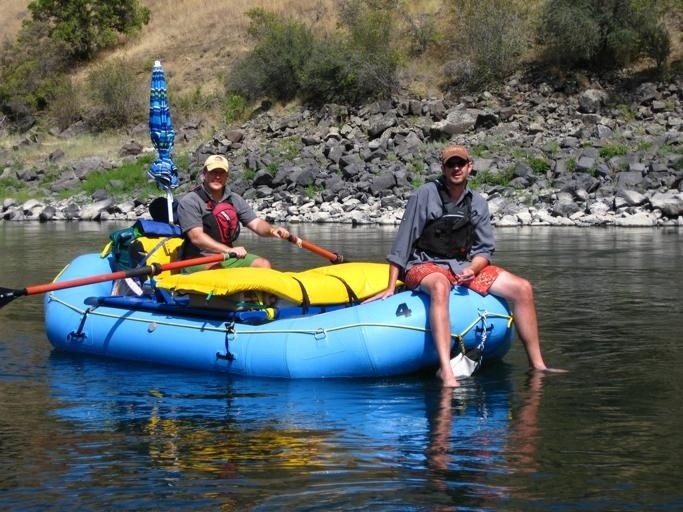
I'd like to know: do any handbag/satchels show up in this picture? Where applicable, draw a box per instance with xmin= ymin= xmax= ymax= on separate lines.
xmin=416 ymin=215 xmax=472 ymax=261
xmin=205 ymin=203 xmax=240 ymax=244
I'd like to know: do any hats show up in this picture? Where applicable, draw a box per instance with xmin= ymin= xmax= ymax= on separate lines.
xmin=203 ymin=155 xmax=229 ymax=174
xmin=441 ymin=146 xmax=468 ymax=165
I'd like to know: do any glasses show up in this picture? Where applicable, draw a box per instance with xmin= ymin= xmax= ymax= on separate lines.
xmin=446 ymin=161 xmax=467 ymax=168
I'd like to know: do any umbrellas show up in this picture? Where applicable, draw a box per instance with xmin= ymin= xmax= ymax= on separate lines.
xmin=146 ymin=60 xmax=180 ymax=223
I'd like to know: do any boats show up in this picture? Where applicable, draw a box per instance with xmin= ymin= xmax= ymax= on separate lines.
xmin=43 ymin=254 xmax=517 ymax=380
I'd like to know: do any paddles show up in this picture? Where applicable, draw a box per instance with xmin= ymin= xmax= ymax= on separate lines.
xmin=0 ymin=253 xmax=238 ymax=308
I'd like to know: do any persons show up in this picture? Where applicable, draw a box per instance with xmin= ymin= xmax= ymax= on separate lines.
xmin=432 ymin=372 xmax=548 ymax=473
xmin=358 ymin=143 xmax=569 ymax=389
xmin=176 ymin=156 xmax=290 ymax=310
